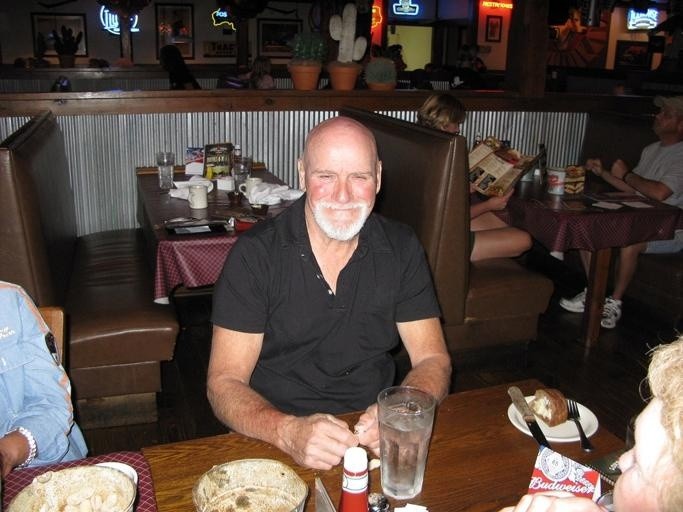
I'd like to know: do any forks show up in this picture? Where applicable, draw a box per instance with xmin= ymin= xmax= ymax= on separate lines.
xmin=565 ymin=398 xmax=594 ymax=453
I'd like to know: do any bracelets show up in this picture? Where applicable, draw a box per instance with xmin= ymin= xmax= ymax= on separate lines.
xmin=623 ymin=171 xmax=634 ymax=184
xmin=4 ymin=427 xmax=36 ymax=467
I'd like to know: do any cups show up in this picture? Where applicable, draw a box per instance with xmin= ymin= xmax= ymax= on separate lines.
xmin=156 ymin=152 xmax=263 ymax=209
xmin=376 ymin=385 xmax=437 ymax=500
xmin=547 ymin=168 xmax=566 ymax=195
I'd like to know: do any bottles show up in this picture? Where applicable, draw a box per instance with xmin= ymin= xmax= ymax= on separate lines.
xmin=339 ymin=446 xmax=370 ymax=512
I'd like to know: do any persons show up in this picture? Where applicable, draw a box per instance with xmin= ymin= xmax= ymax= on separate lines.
xmin=559 ymin=95 xmax=683 ymax=329
xmin=201 ymin=116 xmax=452 ymax=472
xmin=417 ymin=92 xmax=533 ymax=263
xmin=497 ymin=329 xmax=682 ymax=512
xmin=161 ymin=45 xmax=274 ymax=90
xmin=0 ymin=281 xmax=89 ymax=479
xmin=371 ymin=45 xmax=487 ymax=91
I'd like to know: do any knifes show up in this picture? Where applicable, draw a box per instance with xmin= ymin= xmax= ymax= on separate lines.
xmin=506 ymin=385 xmax=552 ymax=451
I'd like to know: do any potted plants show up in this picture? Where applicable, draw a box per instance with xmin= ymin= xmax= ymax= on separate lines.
xmin=53 ymin=26 xmax=82 ymax=68
xmin=288 ymin=31 xmax=327 ymax=90
xmin=362 ymin=57 xmax=398 ymax=90
xmin=327 ymin=4 xmax=368 ymax=90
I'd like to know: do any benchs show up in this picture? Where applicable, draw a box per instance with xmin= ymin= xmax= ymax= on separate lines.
xmin=0 ymin=108 xmax=181 ymax=400
xmin=50 ymin=75 xmax=72 ymax=92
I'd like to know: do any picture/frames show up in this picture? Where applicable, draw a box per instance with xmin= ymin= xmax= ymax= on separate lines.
xmin=31 ymin=11 xmax=89 ymax=58
xmin=486 ymin=15 xmax=503 ymax=42
xmin=615 ymin=40 xmax=652 ymax=70
xmin=156 ymin=3 xmax=195 ymax=60
xmin=256 ymin=17 xmax=303 ymax=58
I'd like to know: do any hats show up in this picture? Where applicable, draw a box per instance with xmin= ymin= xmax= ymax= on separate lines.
xmin=653 ymin=94 xmax=683 ymax=111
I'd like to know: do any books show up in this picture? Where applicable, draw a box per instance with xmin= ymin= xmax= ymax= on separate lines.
xmin=469 ymin=137 xmax=546 ymax=196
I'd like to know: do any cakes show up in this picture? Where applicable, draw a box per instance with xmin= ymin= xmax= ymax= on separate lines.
xmin=527 ymin=387 xmax=569 ymax=429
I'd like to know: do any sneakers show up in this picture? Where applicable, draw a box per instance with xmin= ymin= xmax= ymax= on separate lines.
xmin=601 ymin=297 xmax=624 ymax=330
xmin=559 ymin=287 xmax=588 ymax=315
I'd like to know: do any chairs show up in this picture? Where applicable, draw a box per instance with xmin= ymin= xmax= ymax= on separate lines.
xmin=37 ymin=307 xmax=65 ymax=371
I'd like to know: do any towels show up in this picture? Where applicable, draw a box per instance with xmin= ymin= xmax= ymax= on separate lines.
xmin=248 ymin=183 xmax=305 ymax=206
xmin=168 ymin=174 xmax=214 ymax=202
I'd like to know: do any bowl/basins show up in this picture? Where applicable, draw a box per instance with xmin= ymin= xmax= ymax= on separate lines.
xmin=191 ymin=458 xmax=310 ymax=512
xmin=4 ymin=464 xmax=137 ymax=512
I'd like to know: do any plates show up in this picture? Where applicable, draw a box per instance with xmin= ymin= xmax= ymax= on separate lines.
xmin=95 ymin=461 xmax=138 ymax=486
xmin=507 ymin=395 xmax=600 ymax=443
